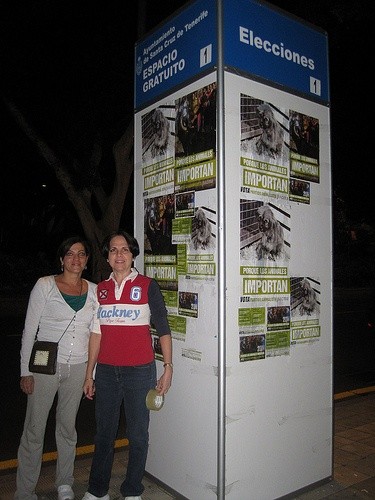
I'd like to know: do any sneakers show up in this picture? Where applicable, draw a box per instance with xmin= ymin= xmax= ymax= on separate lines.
xmin=124 ymin=496 xmax=141 ymax=500
xmin=57 ymin=485 xmax=74 ymax=500
xmin=82 ymin=492 xmax=110 ymax=500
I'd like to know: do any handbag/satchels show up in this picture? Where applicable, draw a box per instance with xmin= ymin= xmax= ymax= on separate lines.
xmin=28 ymin=340 xmax=58 ymax=375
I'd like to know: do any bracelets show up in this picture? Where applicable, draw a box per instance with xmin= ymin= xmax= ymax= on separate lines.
xmin=164 ymin=362 xmax=174 ymax=367
xmin=85 ymin=377 xmax=93 ymax=380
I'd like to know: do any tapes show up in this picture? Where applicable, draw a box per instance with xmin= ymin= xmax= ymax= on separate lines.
xmin=146 ymin=389 xmax=165 ymax=411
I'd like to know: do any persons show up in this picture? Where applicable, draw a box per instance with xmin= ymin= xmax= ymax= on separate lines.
xmin=83 ymin=230 xmax=175 ymax=500
xmin=14 ymin=234 xmax=99 ymax=500
